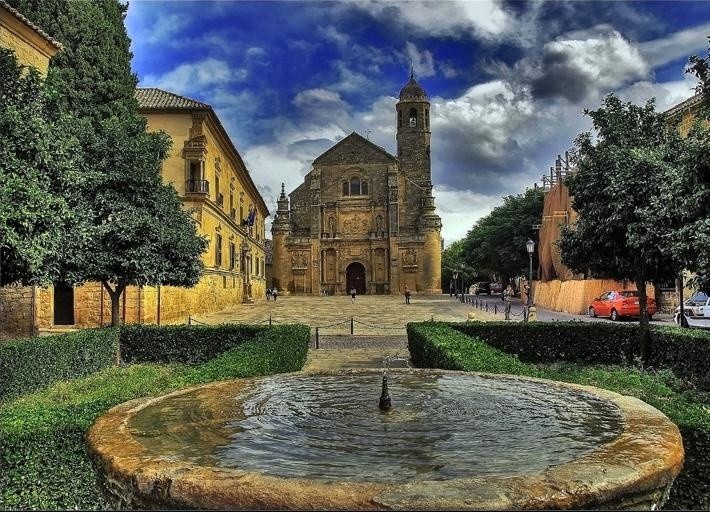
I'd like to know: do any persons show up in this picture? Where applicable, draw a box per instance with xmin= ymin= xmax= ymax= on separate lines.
xmin=503 ymin=284 xmax=515 ymax=320
xmin=404 ymin=285 xmax=412 ymax=304
xmin=350 ymin=286 xmax=357 ymax=305
xmin=266 ymin=287 xmax=278 ymax=302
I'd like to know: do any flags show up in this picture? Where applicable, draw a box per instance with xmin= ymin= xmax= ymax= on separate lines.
xmin=248 ymin=211 xmax=256 ymax=226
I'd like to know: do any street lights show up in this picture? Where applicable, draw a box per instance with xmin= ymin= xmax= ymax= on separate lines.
xmin=525 ymin=237 xmax=536 ymax=310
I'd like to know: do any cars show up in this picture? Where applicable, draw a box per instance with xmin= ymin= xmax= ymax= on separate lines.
xmin=587 ymin=289 xmax=656 ymax=322
xmin=673 ymin=290 xmax=710 ymax=331
